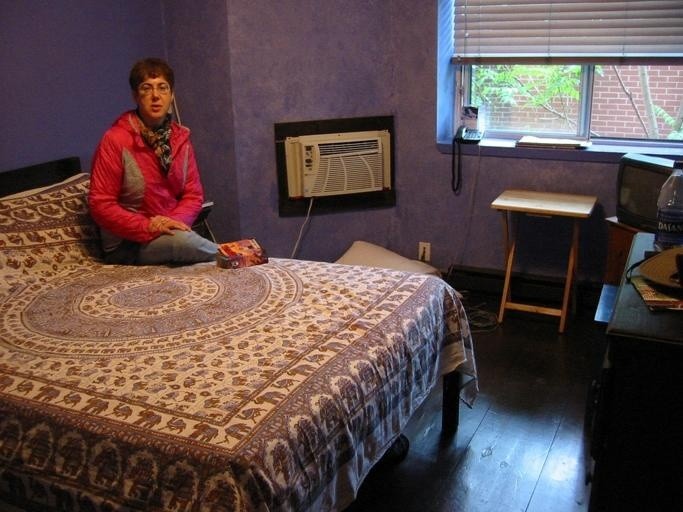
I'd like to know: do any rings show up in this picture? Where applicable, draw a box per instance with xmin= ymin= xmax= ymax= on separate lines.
xmin=160 ymin=217 xmax=168 ymax=222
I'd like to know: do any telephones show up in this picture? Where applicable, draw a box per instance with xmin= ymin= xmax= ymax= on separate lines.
xmin=456 ymin=126 xmax=483 ymax=144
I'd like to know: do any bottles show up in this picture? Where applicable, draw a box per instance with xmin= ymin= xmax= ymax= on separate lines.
xmin=653 ymin=160 xmax=683 ymax=249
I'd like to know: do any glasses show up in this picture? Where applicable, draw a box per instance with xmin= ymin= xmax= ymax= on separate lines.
xmin=138 ymin=84 xmax=172 ymax=95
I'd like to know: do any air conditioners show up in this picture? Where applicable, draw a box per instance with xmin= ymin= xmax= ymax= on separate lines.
xmin=286 ymin=131 xmax=392 ymax=199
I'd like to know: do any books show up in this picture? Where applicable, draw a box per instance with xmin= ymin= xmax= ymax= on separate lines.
xmin=516 ymin=135 xmax=594 ymax=151
xmin=629 ymin=276 xmax=683 ymax=312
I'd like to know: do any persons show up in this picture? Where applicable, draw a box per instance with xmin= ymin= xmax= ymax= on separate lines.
xmin=89 ymin=57 xmax=219 ymax=267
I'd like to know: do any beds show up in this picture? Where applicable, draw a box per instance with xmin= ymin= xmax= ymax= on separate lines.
xmin=0 ymin=156 xmax=480 ymax=512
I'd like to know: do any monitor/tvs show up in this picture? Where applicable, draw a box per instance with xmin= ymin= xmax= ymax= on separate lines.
xmin=617 ymin=154 xmax=676 ymax=235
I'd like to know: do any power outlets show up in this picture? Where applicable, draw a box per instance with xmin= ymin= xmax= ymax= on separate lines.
xmin=417 ymin=242 xmax=430 ymax=261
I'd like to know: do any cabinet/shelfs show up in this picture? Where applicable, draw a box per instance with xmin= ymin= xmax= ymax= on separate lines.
xmin=582 ymin=234 xmax=683 ymax=512
xmin=605 ymin=216 xmax=638 ymax=289
